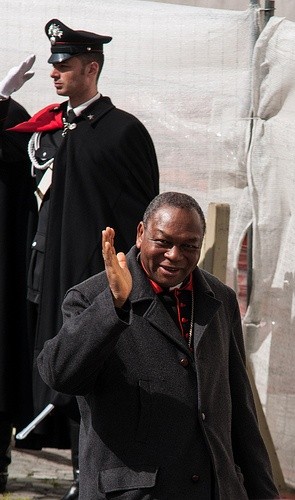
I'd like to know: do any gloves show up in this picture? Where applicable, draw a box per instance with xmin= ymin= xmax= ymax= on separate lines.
xmin=0 ymin=54 xmax=36 ymax=102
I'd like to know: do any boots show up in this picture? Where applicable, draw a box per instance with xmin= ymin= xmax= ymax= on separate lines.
xmin=61 ymin=454 xmax=79 ymax=500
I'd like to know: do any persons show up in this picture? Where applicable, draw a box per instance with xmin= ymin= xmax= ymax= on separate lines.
xmin=34 ymin=192 xmax=279 ymax=500
xmin=0 ymin=18 xmax=160 ymax=500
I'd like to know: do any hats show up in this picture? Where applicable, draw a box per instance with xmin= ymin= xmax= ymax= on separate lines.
xmin=45 ymin=19 xmax=112 ymax=63
